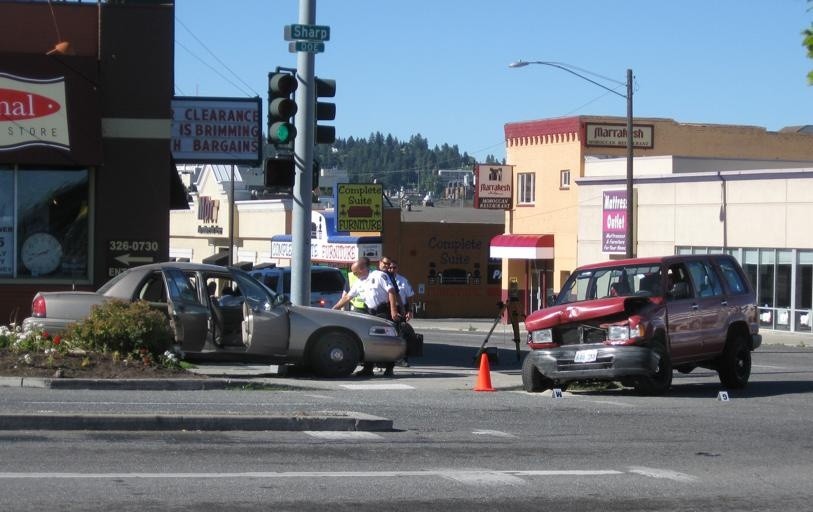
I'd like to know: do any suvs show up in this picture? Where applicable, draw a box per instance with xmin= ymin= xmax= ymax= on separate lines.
xmin=520 ymin=254 xmax=761 ymax=395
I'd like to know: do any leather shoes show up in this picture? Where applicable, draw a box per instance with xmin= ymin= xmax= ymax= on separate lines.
xmin=355 ymin=368 xmax=394 ymax=377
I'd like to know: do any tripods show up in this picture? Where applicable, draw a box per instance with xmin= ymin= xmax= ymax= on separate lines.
xmin=470 ymin=296 xmax=526 ymax=366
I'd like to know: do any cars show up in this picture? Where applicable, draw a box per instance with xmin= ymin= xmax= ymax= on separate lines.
xmin=248 ymin=265 xmax=351 ymax=311
xmin=20 ymin=262 xmax=407 ymax=380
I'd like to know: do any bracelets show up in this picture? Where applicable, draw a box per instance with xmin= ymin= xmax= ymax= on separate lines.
xmin=407 ymin=308 xmax=412 ymax=312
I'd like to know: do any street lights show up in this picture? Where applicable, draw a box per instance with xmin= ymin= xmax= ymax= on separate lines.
xmin=508 ymin=59 xmax=633 ymax=259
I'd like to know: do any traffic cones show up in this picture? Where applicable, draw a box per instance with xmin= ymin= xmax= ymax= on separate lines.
xmin=473 ymin=353 xmax=496 ymax=392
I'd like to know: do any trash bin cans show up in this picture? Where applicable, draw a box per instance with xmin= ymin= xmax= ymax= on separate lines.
xmin=412 ymin=303 xmax=425 ymax=319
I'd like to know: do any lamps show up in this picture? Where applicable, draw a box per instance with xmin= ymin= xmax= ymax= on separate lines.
xmin=45 ymin=0 xmax=69 ymax=57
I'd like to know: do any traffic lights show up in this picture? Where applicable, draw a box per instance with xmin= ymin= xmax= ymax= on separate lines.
xmin=267 ymin=72 xmax=297 ymax=145
xmin=315 ymin=75 xmax=335 ymax=145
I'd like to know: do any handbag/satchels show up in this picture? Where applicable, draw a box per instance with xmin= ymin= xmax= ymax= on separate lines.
xmin=399 ymin=321 xmax=424 ymax=356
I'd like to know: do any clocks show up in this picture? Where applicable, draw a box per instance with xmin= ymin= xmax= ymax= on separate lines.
xmin=20 ymin=232 xmax=63 ymax=276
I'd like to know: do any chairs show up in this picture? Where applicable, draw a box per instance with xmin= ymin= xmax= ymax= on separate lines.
xmin=210 ymin=295 xmax=245 ymax=348
xmin=148 ymin=277 xmax=164 ymax=302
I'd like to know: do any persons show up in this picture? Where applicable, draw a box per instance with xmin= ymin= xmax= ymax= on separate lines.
xmin=217 ymin=285 xmax=244 ymax=306
xmin=374 ymin=256 xmax=407 ymax=324
xmin=387 ymin=260 xmax=414 ymax=321
xmin=329 ymin=259 xmax=402 ymax=377
xmin=340 ymin=257 xmax=371 ymax=314
